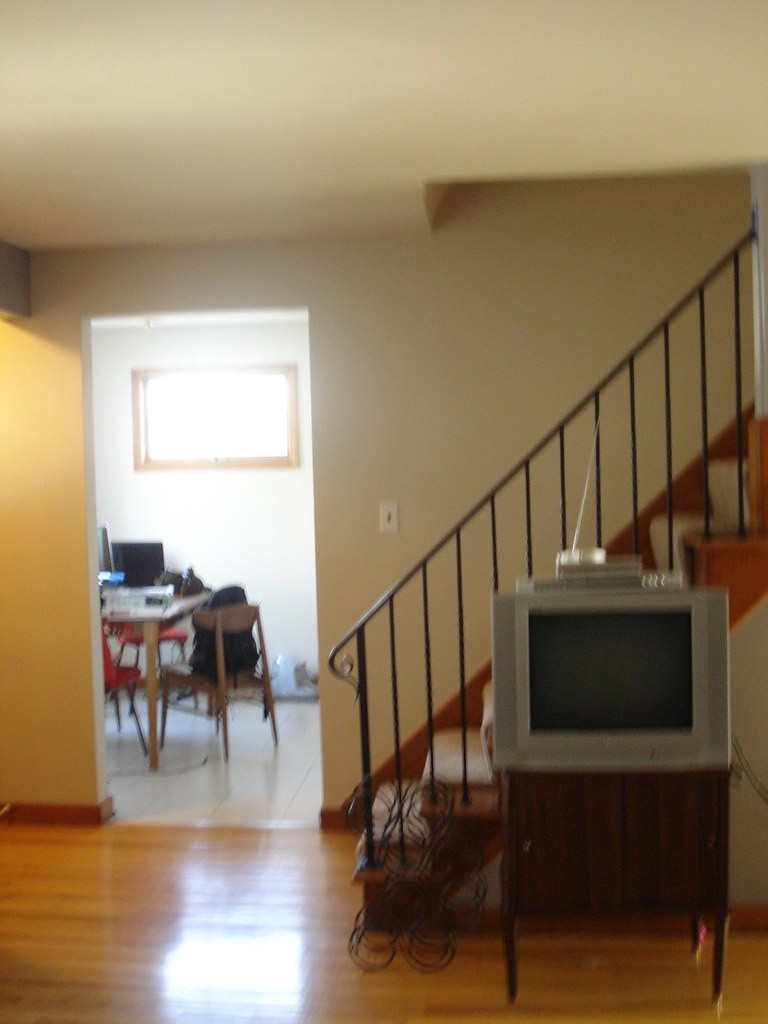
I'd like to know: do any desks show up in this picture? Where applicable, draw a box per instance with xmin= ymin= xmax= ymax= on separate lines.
xmin=99 ymin=585 xmax=214 ymax=772
xmin=496 ymin=763 xmax=732 ymax=1010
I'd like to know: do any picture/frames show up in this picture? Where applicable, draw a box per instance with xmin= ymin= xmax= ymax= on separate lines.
xmin=130 ymin=362 xmax=298 ymax=472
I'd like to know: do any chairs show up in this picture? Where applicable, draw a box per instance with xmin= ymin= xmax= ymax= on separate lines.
xmin=99 ymin=585 xmax=281 ymax=764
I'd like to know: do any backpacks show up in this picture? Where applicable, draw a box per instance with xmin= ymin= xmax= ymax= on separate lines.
xmin=190 ymin=584 xmax=258 ymax=679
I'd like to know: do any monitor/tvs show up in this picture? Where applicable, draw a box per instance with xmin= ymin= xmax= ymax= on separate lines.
xmin=491 ymin=588 xmax=732 ymax=773
xmin=98 ymin=527 xmax=165 ymax=586
